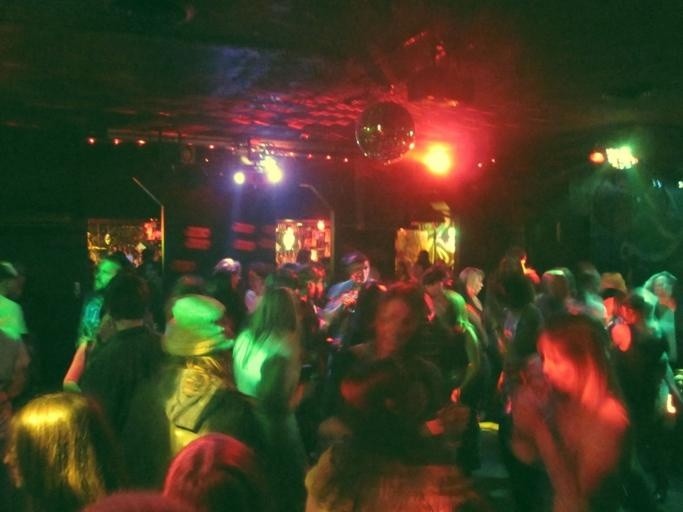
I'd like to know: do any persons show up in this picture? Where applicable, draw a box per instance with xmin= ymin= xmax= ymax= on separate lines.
xmin=1 ymin=247 xmax=683 ymax=512
